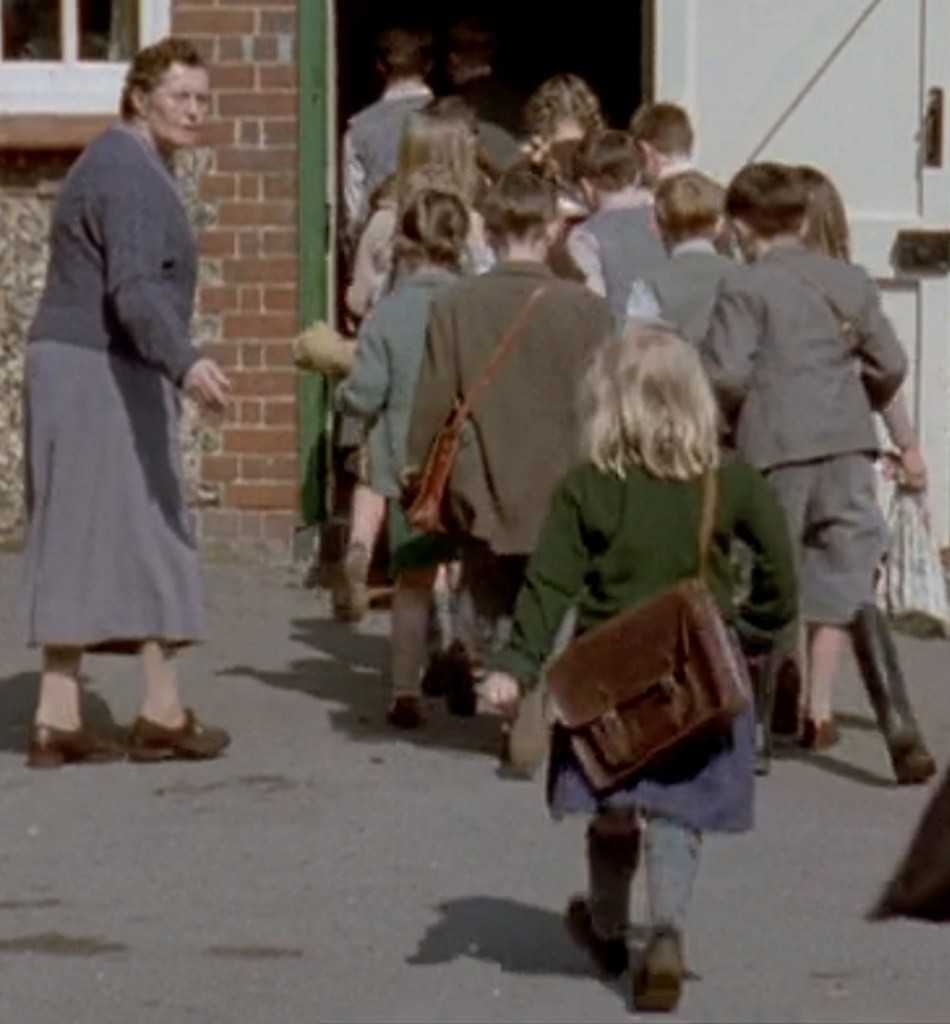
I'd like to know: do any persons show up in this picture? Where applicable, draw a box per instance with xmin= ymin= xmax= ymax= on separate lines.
xmin=400 ymin=170 xmax=613 ymax=774
xmin=626 ymin=174 xmax=802 ymax=742
xmin=331 ymin=192 xmax=474 ymax=728
xmin=794 ymin=165 xmax=928 ymax=493
xmin=332 ymin=24 xmax=730 ymax=620
xmin=25 ymin=38 xmax=232 ymax=764
xmin=701 ymin=164 xmax=909 ymax=747
xmin=479 ymin=331 xmax=799 ymax=1010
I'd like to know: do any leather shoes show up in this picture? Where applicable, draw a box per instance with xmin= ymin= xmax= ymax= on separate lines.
xmin=28 ymin=718 xmax=123 ymax=767
xmin=127 ymin=707 xmax=229 ymax=761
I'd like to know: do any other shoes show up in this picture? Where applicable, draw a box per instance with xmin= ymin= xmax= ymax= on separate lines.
xmin=568 ymin=894 xmax=631 ymax=976
xmin=633 ymin=923 xmax=683 ymax=1013
xmin=420 ymin=637 xmax=471 ymax=697
xmin=384 ymin=696 xmax=422 ymax=729
xmin=332 ymin=541 xmax=367 ymax=622
xmin=805 ymin=712 xmax=837 ymax=752
xmin=769 ymin=656 xmax=800 ymax=738
xmin=445 ymin=661 xmax=478 ymax=718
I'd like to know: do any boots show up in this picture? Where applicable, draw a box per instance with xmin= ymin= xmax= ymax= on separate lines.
xmin=844 ymin=597 xmax=936 ymax=782
xmin=746 ymin=655 xmax=775 ymax=775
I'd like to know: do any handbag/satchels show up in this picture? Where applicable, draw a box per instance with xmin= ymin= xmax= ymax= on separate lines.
xmin=401 ymin=427 xmax=466 ymax=536
xmin=545 ymin=578 xmax=755 ymax=794
xmin=875 ymin=453 xmax=950 ymax=641
